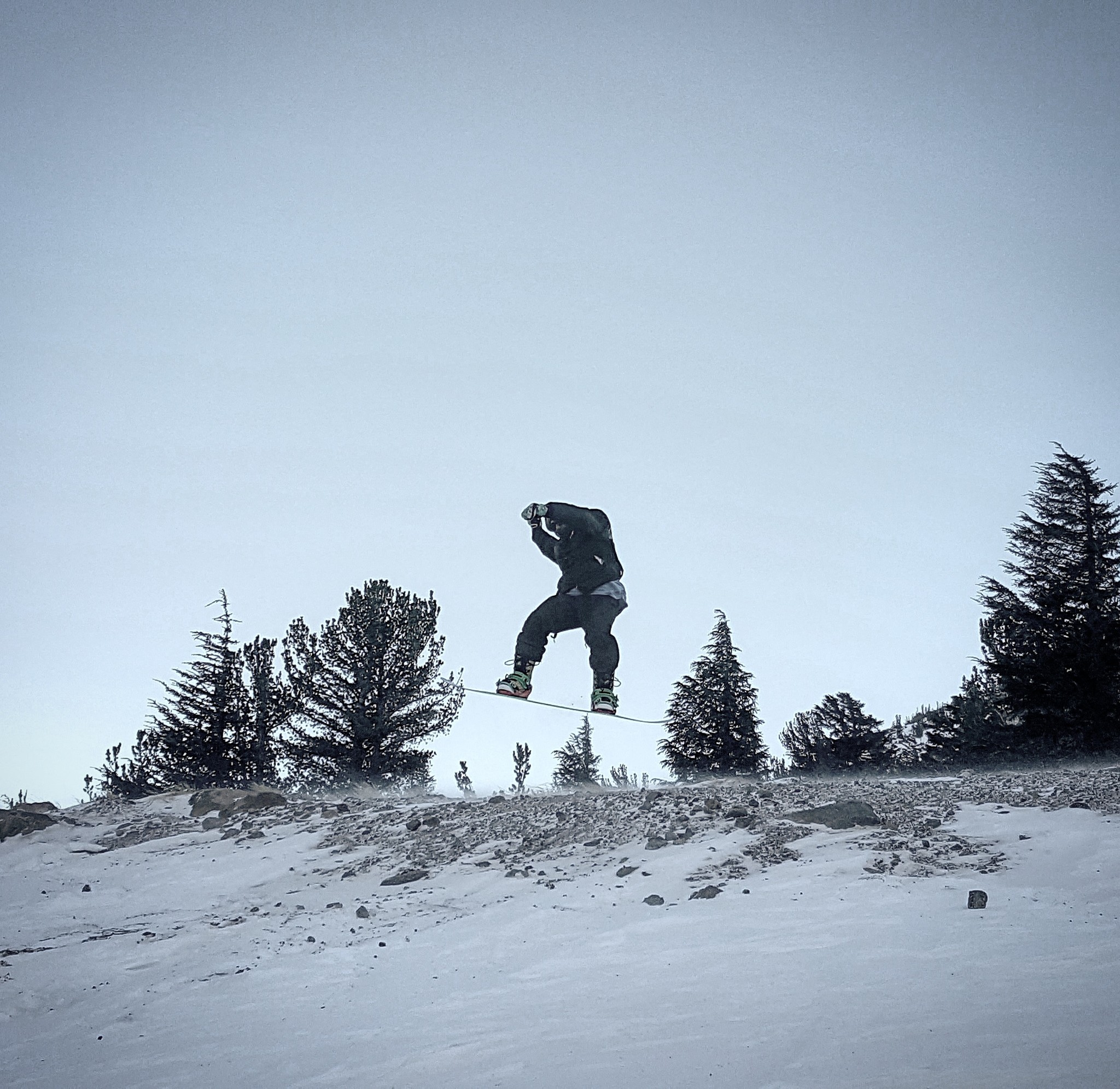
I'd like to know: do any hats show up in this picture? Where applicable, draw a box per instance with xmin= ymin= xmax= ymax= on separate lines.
xmin=545 ymin=517 xmax=558 ymax=532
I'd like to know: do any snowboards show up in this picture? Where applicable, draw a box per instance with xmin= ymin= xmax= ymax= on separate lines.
xmin=440 ymin=677 xmax=675 ymax=723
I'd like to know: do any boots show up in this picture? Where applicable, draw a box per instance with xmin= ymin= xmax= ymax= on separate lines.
xmin=591 ymin=670 xmax=621 ymax=715
xmin=496 ymin=655 xmax=540 ymax=698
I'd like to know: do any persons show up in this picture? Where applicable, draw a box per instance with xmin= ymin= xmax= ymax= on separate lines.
xmin=496 ymin=502 xmax=628 ymax=715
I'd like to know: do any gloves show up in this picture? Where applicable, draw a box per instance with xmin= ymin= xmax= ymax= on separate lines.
xmin=521 ymin=503 xmax=548 ymax=520
xmin=527 ymin=518 xmax=543 ymax=529
